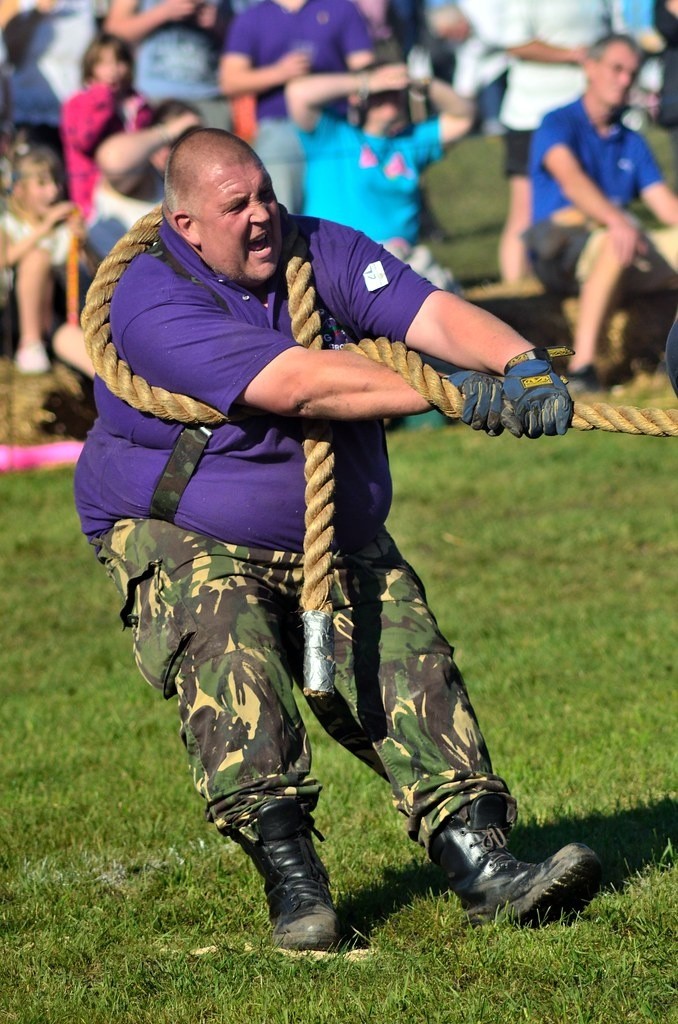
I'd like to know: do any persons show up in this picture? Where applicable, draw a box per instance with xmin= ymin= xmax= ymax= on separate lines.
xmin=71 ymin=125 xmax=610 ymax=950
xmin=2 ymin=1 xmax=677 ymax=389
xmin=518 ymin=36 xmax=678 ymax=390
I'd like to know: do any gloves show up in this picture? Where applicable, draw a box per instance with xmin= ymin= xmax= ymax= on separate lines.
xmin=504 ymin=345 xmax=578 ymax=438
xmin=449 ymin=368 xmax=506 ymax=436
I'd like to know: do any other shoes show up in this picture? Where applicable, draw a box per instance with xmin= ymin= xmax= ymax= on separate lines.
xmin=567 ymin=365 xmax=602 ymax=394
xmin=17 ymin=339 xmax=51 ymax=375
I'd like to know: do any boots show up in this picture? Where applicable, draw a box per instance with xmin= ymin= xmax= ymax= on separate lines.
xmin=229 ymin=799 xmax=339 ymax=949
xmin=427 ymin=794 xmax=600 ymax=933
